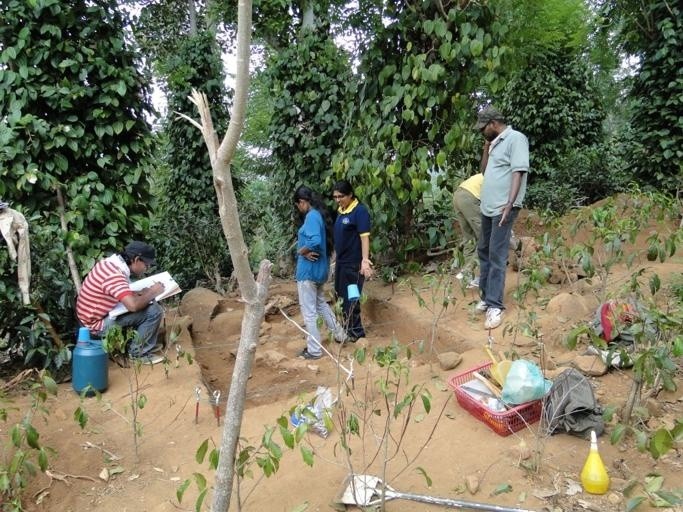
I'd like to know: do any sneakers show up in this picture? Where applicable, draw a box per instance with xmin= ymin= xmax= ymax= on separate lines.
xmin=149 ymin=343 xmax=163 ymax=352
xmin=484 ymin=307 xmax=503 ymax=329
xmin=455 ymin=271 xmax=480 ymax=288
xmin=300 ymin=349 xmax=321 ymax=359
xmin=335 ymin=336 xmax=358 ymax=342
xmin=128 ymin=354 xmax=164 ymax=364
xmin=476 ymin=301 xmax=488 ymax=311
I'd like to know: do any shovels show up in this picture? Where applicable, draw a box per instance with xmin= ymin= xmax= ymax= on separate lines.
xmin=332 ymin=473 xmax=537 ymax=512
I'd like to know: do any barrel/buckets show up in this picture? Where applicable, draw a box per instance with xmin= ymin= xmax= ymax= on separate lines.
xmin=72 ymin=327 xmax=108 ymax=397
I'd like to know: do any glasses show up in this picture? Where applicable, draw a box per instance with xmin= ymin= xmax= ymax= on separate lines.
xmin=333 ymin=195 xmax=345 ymax=198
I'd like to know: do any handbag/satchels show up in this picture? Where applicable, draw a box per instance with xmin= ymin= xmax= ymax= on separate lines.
xmin=545 ymin=367 xmax=604 ymax=441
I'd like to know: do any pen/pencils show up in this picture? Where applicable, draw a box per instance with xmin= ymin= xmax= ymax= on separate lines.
xmin=153 ymin=280 xmax=156 ymax=284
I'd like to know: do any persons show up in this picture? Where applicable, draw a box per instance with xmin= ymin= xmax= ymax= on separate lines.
xmin=329 ymin=179 xmax=374 ymax=344
xmin=74 ymin=241 xmax=166 ymax=364
xmin=292 ymin=185 xmax=348 ymax=359
xmin=472 ymin=106 xmax=529 ymax=330
xmin=452 ymin=171 xmax=522 ymax=291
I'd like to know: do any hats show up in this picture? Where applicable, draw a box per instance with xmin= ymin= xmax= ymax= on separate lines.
xmin=473 ymin=107 xmax=505 ymax=130
xmin=126 ymin=241 xmax=158 ymax=266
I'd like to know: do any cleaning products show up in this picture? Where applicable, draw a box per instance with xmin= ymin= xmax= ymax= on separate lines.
xmin=579 ymin=430 xmax=610 ymax=496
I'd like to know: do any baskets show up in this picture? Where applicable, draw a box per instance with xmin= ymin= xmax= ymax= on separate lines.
xmin=450 ymin=361 xmax=547 ymax=436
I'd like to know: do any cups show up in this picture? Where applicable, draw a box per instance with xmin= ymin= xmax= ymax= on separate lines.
xmin=77 ymin=327 xmax=90 ymax=343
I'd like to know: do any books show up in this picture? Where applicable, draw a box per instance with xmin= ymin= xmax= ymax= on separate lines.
xmin=107 ymin=271 xmax=182 ymax=317
